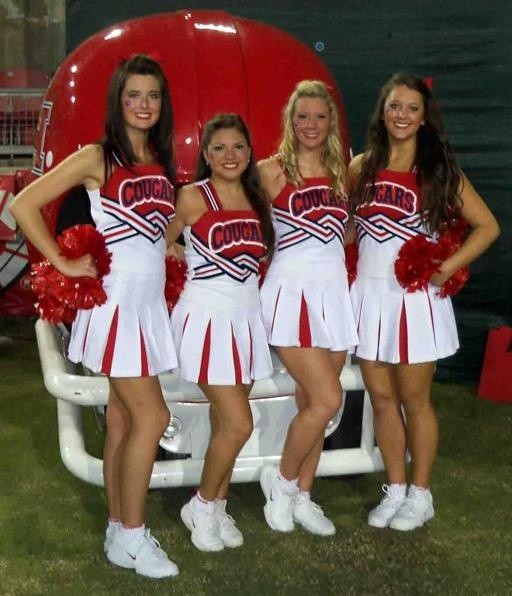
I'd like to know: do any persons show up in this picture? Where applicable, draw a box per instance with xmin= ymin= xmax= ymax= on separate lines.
xmin=343 ymin=69 xmax=502 ymax=533
xmin=161 ymin=111 xmax=275 ymax=555
xmin=7 ymin=52 xmax=187 ymax=580
xmin=255 ymin=79 xmax=363 ymax=538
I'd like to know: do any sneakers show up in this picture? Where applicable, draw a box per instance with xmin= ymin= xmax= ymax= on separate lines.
xmin=292 ymin=494 xmax=335 ymax=535
xmin=180 ymin=499 xmax=244 ymax=552
xmin=367 ymin=494 xmax=434 ymax=530
xmin=259 ymin=466 xmax=294 ymax=532
xmin=104 ymin=527 xmax=179 ymax=578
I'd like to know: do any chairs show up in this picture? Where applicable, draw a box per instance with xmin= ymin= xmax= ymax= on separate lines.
xmin=0 ymin=65 xmax=52 ymax=149
